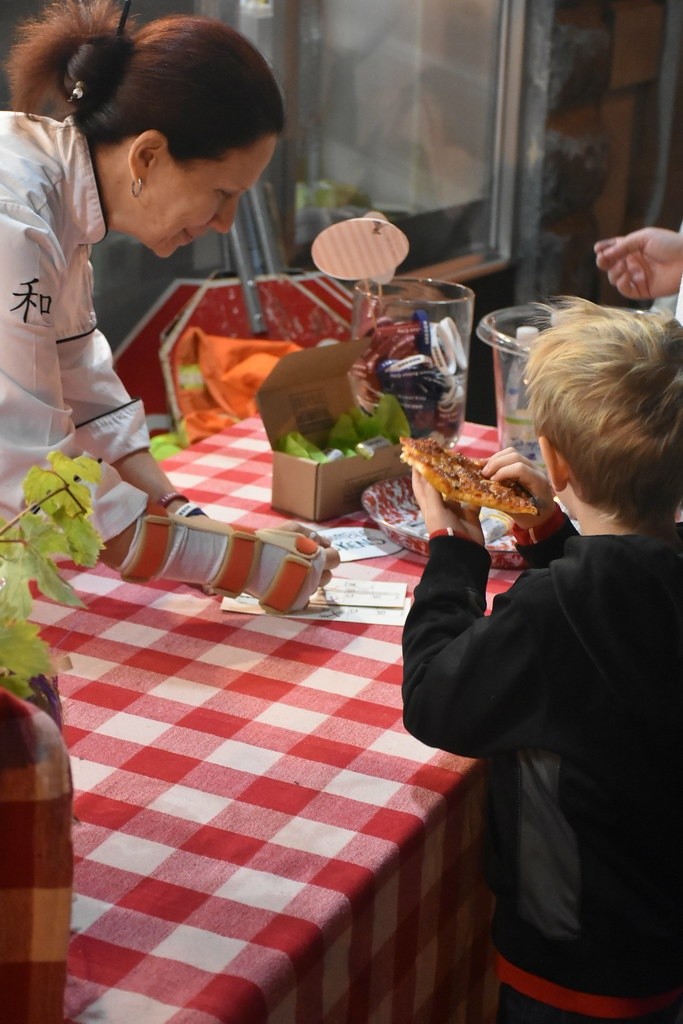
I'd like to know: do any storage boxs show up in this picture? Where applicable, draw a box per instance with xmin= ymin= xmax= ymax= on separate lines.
xmin=255 ymin=336 xmax=414 ymax=522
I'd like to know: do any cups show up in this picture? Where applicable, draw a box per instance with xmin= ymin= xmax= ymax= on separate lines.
xmin=477 ymin=303 xmax=556 ymax=475
xmin=355 ymin=278 xmax=474 ymax=446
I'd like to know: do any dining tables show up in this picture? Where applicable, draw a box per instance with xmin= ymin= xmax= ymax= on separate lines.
xmin=29 ymin=413 xmax=599 ymax=1024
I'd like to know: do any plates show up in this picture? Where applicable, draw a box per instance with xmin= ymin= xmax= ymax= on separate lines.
xmin=362 ymin=474 xmax=536 ymax=568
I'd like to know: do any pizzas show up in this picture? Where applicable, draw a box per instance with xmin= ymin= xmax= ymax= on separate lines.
xmin=400 ymin=439 xmax=539 ymax=516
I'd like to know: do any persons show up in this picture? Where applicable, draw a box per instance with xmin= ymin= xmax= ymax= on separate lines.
xmin=403 ymin=301 xmax=683 ymax=1024
xmin=594 ymin=226 xmax=683 ymax=301
xmin=0 ymin=0 xmax=339 ymax=613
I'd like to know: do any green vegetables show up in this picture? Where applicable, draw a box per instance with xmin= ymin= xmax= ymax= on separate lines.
xmin=286 ymin=396 xmax=409 ymax=464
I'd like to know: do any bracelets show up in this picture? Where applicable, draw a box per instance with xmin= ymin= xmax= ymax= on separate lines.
xmin=159 ymin=492 xmax=206 ymax=517
